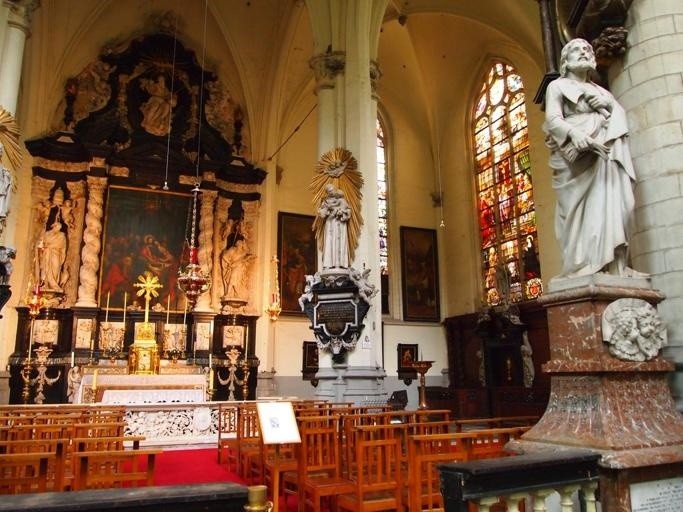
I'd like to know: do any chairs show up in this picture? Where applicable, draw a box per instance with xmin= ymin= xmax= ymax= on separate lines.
xmin=0 ymin=408 xmax=165 ymax=496
xmin=217 ymin=399 xmax=540 ymax=512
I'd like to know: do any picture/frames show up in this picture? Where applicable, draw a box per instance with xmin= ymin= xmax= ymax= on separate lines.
xmin=395 ymin=343 xmax=419 ymax=386
xmin=398 ymin=224 xmax=442 ymax=323
xmin=300 ymin=339 xmax=320 ymax=387
xmin=276 ymin=209 xmax=316 ymax=317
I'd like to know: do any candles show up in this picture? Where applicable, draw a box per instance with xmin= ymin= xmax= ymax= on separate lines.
xmin=70 ymin=350 xmax=75 ymax=367
xmin=244 ymin=334 xmax=248 ymax=362
xmin=91 ymin=338 xmax=94 ymax=350
xmin=182 ymin=298 xmax=188 ymax=330
xmin=105 ymin=289 xmax=111 ymax=326
xmin=91 ymin=369 xmax=97 ymax=389
xmin=123 ymin=290 xmax=128 ymax=329
xmin=165 ymin=293 xmax=171 ymax=329
xmin=210 ymin=368 xmax=214 ymax=389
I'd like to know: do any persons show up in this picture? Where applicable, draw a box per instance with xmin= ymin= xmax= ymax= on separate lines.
xmin=145 ymin=76 xmax=172 ymax=128
xmin=541 ymin=38 xmax=652 ymax=281
xmin=221 ymin=240 xmax=252 ymax=298
xmin=41 ymin=222 xmax=67 ymax=290
xmin=69 ymin=366 xmax=81 ymax=401
xmin=318 ymin=184 xmax=352 ymax=270
xmin=328 ymin=189 xmax=347 ymax=218
xmin=220 ymin=218 xmax=238 ymax=241
xmin=57 ymin=200 xmax=76 ymax=229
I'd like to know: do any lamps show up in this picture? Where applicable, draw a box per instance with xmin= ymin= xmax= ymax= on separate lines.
xmin=173 ymin=0 xmax=214 ymax=307
xmin=264 ymin=253 xmax=282 ymax=322
